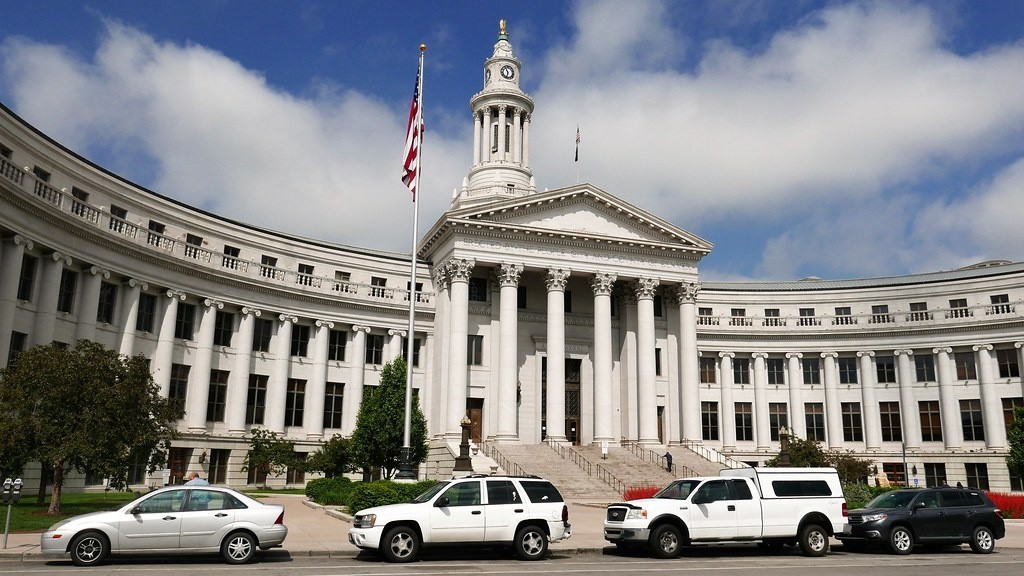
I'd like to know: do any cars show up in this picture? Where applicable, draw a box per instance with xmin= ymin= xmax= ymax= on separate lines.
xmin=41 ymin=486 xmax=287 ymax=567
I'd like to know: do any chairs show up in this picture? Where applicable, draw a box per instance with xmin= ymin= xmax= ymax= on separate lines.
xmin=457 ymin=487 xmax=473 ymax=505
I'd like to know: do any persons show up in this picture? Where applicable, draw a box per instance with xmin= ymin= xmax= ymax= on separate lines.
xmin=957 ymin=482 xmax=963 ymax=490
xmin=177 ymin=472 xmax=213 ymax=511
xmin=661 ymin=451 xmax=672 ymax=473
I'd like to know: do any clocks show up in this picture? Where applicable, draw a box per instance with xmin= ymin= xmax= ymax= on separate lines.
xmin=499 ymin=64 xmax=514 ymax=80
xmin=484 ymin=69 xmax=491 ymax=82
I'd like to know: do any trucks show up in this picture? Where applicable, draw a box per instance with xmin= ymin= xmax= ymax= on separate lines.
xmin=603 ymin=466 xmax=851 ymax=559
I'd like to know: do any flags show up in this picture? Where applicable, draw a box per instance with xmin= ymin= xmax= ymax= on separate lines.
xmin=575 ymin=125 xmax=580 ymax=161
xmin=401 ymin=53 xmax=425 ymax=201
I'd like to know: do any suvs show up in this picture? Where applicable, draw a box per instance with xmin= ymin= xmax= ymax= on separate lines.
xmin=837 ymin=486 xmax=1005 ymax=554
xmin=348 ymin=474 xmax=572 ymax=563
